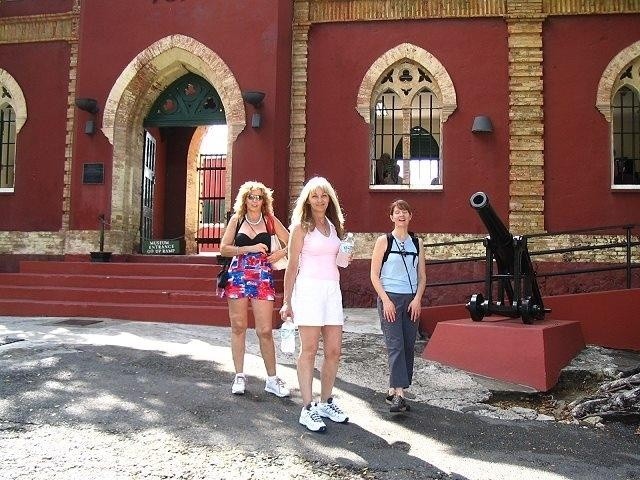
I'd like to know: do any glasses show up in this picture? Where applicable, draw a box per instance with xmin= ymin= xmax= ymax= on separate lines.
xmin=247 ymin=194 xmax=263 ymax=201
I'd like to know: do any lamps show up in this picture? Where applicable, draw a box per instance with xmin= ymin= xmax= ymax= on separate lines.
xmin=75 ymin=95 xmax=100 ymax=116
xmin=241 ymin=88 xmax=267 ymax=112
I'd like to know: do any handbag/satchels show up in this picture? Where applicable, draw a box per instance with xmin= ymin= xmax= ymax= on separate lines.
xmin=270 ymin=234 xmax=288 ymax=271
xmin=216 ymin=269 xmax=229 ymax=288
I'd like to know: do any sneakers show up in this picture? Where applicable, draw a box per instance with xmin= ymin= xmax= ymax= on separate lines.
xmin=316 ymin=398 xmax=348 ymax=423
xmin=231 ymin=373 xmax=246 ymax=394
xmin=386 ymin=394 xmax=410 ymax=412
xmin=299 ymin=402 xmax=327 ymax=432
xmin=264 ymin=375 xmax=290 ymax=397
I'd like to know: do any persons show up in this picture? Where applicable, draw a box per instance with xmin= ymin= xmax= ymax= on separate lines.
xmin=279 ymin=176 xmax=353 ymax=433
xmin=376 ymin=160 xmax=384 ymax=185
xmin=219 ymin=181 xmax=291 ymax=398
xmin=384 ymin=166 xmax=402 ymax=185
xmin=370 ymin=200 xmax=427 ymax=413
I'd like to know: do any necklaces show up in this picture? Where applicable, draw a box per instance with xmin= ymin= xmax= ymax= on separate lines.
xmin=312 ymin=216 xmax=329 ymax=236
xmin=393 ymin=176 xmax=398 ymax=184
xmin=245 ymin=212 xmax=263 ymax=226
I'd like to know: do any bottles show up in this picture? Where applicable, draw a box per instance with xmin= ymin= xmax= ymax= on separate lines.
xmin=335 ymin=231 xmax=355 ymax=268
xmin=281 ymin=315 xmax=296 ymax=355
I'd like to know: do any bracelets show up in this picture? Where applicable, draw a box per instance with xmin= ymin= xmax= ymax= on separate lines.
xmin=283 ymin=302 xmax=289 ymax=305
xmin=244 ymin=245 xmax=248 ymax=256
xmin=236 ymin=244 xmax=239 ymax=257
